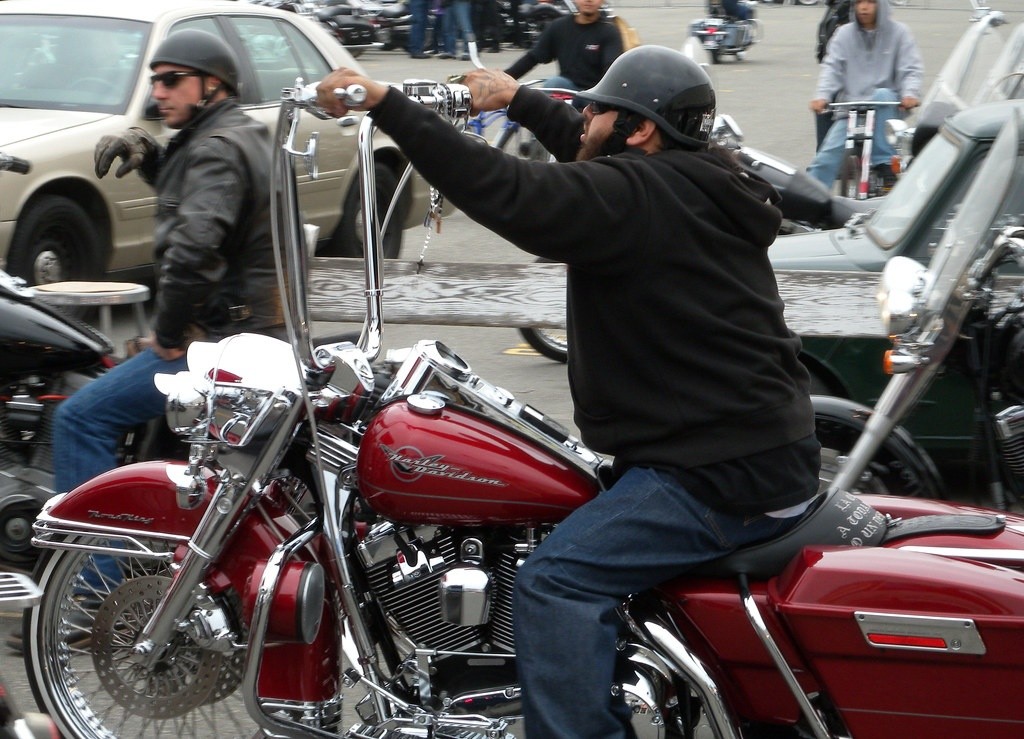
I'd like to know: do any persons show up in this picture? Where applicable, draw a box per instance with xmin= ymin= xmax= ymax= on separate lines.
xmin=316 ymin=44 xmax=823 ymax=739
xmin=408 ymin=0 xmax=499 ymax=60
xmin=43 ymin=30 xmax=287 ymax=653
xmin=494 ymin=0 xmax=625 ymax=92
xmin=708 ymin=0 xmax=753 ymax=25
xmin=807 ymin=1 xmax=924 ymax=188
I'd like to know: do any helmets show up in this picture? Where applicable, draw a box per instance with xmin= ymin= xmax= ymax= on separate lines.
xmin=149 ymin=29 xmax=240 ymax=98
xmin=570 ymin=43 xmax=718 ymax=154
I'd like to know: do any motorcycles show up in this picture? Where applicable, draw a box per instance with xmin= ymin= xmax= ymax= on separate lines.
xmin=20 ymin=45 xmax=1022 ymax=738
xmin=815 ymin=101 xmax=903 ymax=199
xmin=0 ymin=146 xmax=194 ymax=574
xmin=806 ymin=224 xmax=1024 ymax=517
xmin=688 ymin=13 xmax=761 ymax=66
xmin=227 ymin=0 xmax=618 ymax=60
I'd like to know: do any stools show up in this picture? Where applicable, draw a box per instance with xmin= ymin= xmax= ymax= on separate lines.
xmin=31 ymin=281 xmax=151 ymax=339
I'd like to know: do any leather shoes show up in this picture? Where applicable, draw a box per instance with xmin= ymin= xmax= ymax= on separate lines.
xmin=5 ymin=593 xmax=130 ymax=655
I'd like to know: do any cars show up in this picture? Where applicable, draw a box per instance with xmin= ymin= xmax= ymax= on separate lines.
xmin=0 ymin=3 xmax=455 ymax=289
xmin=770 ymin=100 xmax=1024 ymax=485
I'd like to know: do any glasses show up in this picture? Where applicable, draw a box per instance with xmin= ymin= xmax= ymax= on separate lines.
xmin=150 ymin=71 xmax=198 ymax=89
xmin=588 ymin=99 xmax=611 ymax=114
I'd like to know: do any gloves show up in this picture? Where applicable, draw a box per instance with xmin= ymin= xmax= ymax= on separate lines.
xmin=93 ymin=127 xmax=148 ymax=179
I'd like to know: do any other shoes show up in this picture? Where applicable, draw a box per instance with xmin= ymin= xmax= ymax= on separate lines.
xmin=411 ymin=50 xmax=472 ymax=61
